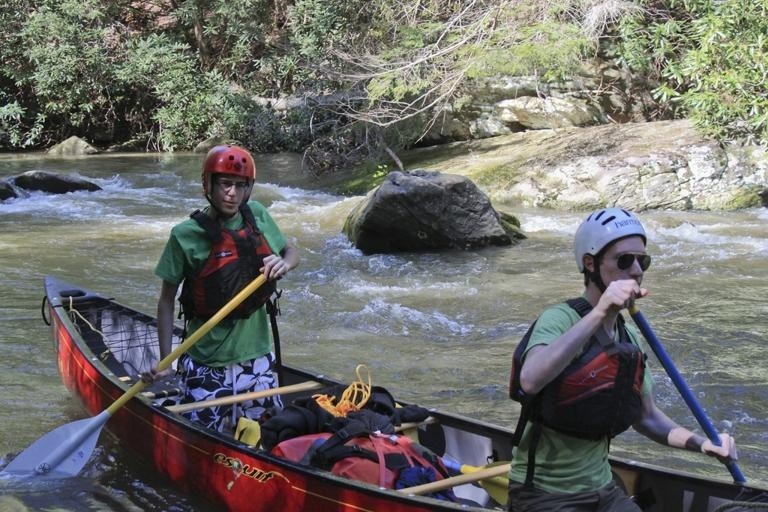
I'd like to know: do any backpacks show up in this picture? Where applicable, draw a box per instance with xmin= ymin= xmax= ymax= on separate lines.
xmin=271 ymin=431 xmax=449 ymax=489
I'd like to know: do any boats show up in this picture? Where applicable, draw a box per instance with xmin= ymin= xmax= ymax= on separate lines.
xmin=42 ymin=275 xmax=768 ymax=511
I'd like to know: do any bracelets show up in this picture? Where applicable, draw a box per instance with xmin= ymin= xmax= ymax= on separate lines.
xmin=686 ymin=434 xmax=706 ymax=453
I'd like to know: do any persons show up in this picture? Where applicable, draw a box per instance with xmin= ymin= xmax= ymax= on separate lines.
xmin=153 ymin=145 xmax=301 ymax=441
xmin=509 ymin=207 xmax=739 ymax=511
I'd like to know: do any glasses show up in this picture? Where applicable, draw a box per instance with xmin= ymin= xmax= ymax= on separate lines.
xmin=601 ymin=251 xmax=652 ymax=271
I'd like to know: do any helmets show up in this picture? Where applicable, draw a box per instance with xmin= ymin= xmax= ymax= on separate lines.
xmin=574 ymin=207 xmax=647 ymax=272
xmin=201 ymin=144 xmax=256 ymax=209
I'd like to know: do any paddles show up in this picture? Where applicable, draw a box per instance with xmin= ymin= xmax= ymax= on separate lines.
xmin=2 ymin=273 xmax=269 ymax=476
xmin=437 ymin=458 xmax=510 ymax=508
